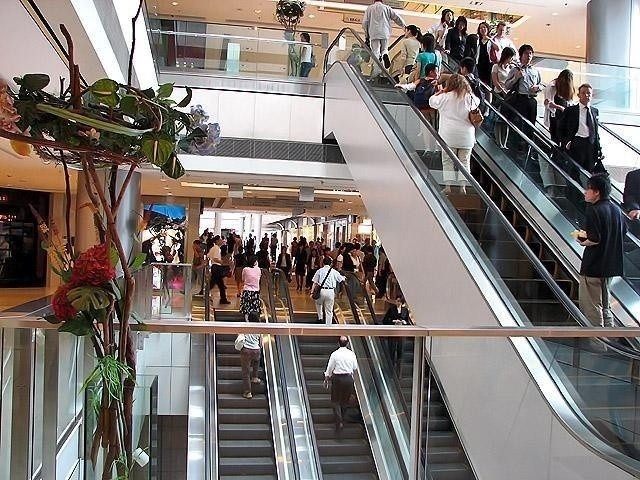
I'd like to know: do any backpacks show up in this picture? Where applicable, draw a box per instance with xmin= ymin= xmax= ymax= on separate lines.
xmin=348 ymin=52 xmax=359 ymax=64
xmin=414 ymin=78 xmax=437 ymax=109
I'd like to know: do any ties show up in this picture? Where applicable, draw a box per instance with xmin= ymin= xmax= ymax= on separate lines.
xmin=585 ymin=106 xmax=595 ymax=144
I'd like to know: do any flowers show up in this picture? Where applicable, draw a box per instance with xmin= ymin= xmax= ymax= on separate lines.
xmin=142 ymin=217 xmax=189 ymax=307
xmin=275 ymin=0 xmax=306 ymax=76
xmin=0 ymin=0 xmax=222 ymax=480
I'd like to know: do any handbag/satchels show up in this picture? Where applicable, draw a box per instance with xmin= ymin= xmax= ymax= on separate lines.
xmin=593 ymin=159 xmax=609 ymax=177
xmin=311 ymin=46 xmax=318 ymax=67
xmin=502 ymin=91 xmax=517 ymax=112
xmin=288 ymin=272 xmax=292 ymax=282
xmin=553 ymin=142 xmax=585 ymax=203
xmin=312 ymin=284 xmax=320 ymax=299
xmin=490 ymin=45 xmax=502 ymax=62
xmin=194 ymin=257 xmax=201 ymax=265
xmin=341 ymin=394 xmax=361 ymax=423
xmin=234 ymin=334 xmax=245 ymax=350
xmin=360 ymin=38 xmax=370 ymax=62
xmin=469 ymin=108 xmax=485 ymax=127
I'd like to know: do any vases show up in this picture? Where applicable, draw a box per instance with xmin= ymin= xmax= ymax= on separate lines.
xmin=158 ymin=281 xmax=173 ymax=314
xmin=84 ymin=375 xmax=159 ymax=479
xmin=287 ymin=54 xmax=300 ymax=76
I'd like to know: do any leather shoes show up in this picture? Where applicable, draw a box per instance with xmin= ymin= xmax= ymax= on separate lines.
xmin=336 ymin=422 xmax=343 ymax=432
xmin=220 ymin=299 xmax=230 ymax=304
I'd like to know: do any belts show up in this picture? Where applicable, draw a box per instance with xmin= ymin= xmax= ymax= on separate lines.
xmin=322 ymin=287 xmax=333 ymax=289
xmin=524 ymin=94 xmax=533 ymax=98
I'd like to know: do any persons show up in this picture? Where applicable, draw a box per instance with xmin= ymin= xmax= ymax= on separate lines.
xmin=575 ymin=174 xmax=629 ymax=354
xmin=362 ymin=0 xmax=406 ymax=81
xmin=194 ymin=229 xmax=409 ymax=399
xmin=539 ymin=68 xmax=601 ymax=198
xmin=435 ymin=9 xmax=541 ymax=149
xmin=322 ymin=336 xmax=358 ymax=433
xmin=623 ymin=168 xmax=640 ymax=220
xmin=300 ymin=33 xmax=313 ymax=77
xmin=398 ymin=25 xmax=484 ymax=200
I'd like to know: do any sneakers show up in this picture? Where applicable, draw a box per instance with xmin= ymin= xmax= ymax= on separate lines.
xmin=443 ymin=187 xmax=451 ymax=192
xmin=252 ymin=377 xmax=261 ymax=383
xmin=243 ymin=392 xmax=252 ymax=398
xmin=383 ymin=54 xmax=390 ymax=68
xmin=460 ymin=187 xmax=466 ymax=194
